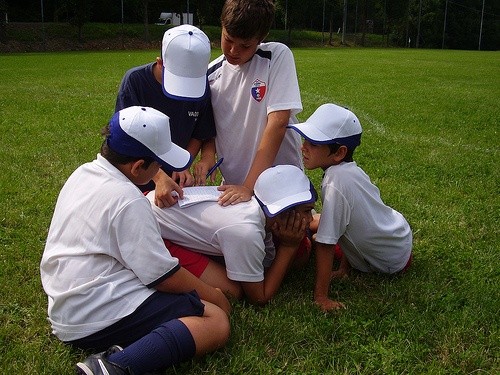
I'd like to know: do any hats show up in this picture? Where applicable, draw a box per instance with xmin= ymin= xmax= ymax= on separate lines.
xmin=160 ymin=23 xmax=210 ymax=100
xmin=107 ymin=104 xmax=192 ymax=173
xmin=252 ymin=163 xmax=320 ymax=215
xmin=287 ymin=101 xmax=364 ymax=147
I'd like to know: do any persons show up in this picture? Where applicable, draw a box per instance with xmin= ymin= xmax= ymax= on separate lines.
xmin=41 ymin=105 xmax=233 ymax=375
xmin=142 ymin=164 xmax=318 ymax=306
xmin=284 ymin=103 xmax=414 ymax=313
xmin=114 ymin=24 xmax=217 ymax=208
xmin=192 ymin=0 xmax=323 ymax=233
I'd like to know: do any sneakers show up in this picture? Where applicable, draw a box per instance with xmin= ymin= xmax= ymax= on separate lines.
xmin=75 ymin=345 xmax=117 ymax=372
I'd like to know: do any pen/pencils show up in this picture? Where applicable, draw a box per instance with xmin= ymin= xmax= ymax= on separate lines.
xmin=205 ymin=157 xmax=224 ymax=179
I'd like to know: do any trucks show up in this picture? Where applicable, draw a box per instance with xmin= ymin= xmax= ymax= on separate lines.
xmin=155 ymin=12 xmax=193 ymax=26
